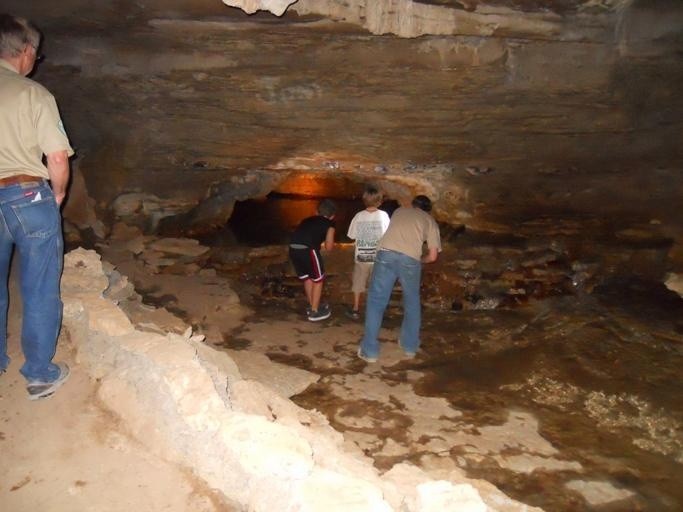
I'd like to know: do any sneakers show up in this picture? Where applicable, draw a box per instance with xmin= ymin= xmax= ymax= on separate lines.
xmin=305 ymin=304 xmax=329 ymax=322
xmin=357 ymin=349 xmax=375 ymax=362
xmin=27 ymin=361 xmax=71 ymax=401
xmin=398 ymin=338 xmax=415 ymax=356
xmin=346 ymin=307 xmax=359 ymax=318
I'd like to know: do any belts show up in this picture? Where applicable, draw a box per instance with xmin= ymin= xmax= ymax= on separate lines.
xmin=0 ymin=174 xmax=43 ymax=189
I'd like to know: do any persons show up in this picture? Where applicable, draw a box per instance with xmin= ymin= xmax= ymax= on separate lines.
xmin=1 ymin=13 xmax=75 ymax=401
xmin=344 ymin=187 xmax=391 ymax=320
xmin=286 ymin=198 xmax=337 ymax=323
xmin=356 ymin=195 xmax=442 ymax=364
